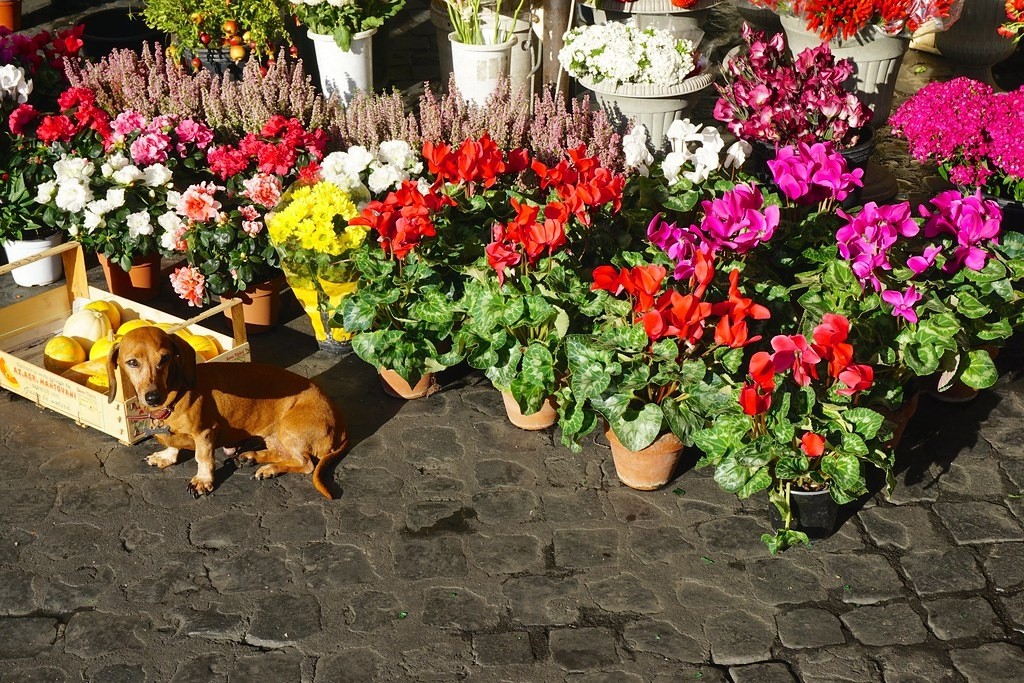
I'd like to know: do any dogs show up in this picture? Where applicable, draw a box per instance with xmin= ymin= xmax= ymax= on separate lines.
xmin=107 ymin=326 xmax=350 ymax=499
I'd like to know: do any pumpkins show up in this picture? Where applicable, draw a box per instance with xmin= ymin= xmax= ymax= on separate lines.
xmin=43 ymin=299 xmax=217 ymax=395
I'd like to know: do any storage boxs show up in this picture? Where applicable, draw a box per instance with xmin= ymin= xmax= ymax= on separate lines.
xmin=0 ymin=241 xmax=252 ymax=446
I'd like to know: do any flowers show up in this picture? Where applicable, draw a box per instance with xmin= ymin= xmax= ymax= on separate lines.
xmin=0 ymin=0 xmax=1024 ymax=554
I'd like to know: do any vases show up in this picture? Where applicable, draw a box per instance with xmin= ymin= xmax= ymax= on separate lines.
xmin=0 ymin=0 xmax=22 ymax=32
xmin=581 ymin=0 xmax=724 ymax=42
xmin=307 ymin=28 xmax=377 ymax=108
xmin=0 ymin=229 xmax=63 ymax=287
xmin=73 ymin=6 xmax=163 ymax=64
xmin=500 ymin=390 xmax=560 ymax=431
xmin=378 ymin=365 xmax=434 ymax=399
xmin=767 ymin=487 xmax=839 ymax=542
xmin=881 ymin=390 xmax=920 ymax=456
xmin=753 ymin=125 xmax=876 ymax=210
xmin=578 ymin=74 xmax=716 ymax=156
xmin=605 ymin=428 xmax=684 ymax=491
xmin=929 ymin=345 xmax=1000 ymax=402
xmin=183 ymin=46 xmax=251 ymax=81
xmin=780 ymin=15 xmax=910 ymax=129
xmin=448 ymin=28 xmax=518 ymax=108
xmin=219 ymin=279 xmax=278 ymax=335
xmin=96 ymin=250 xmax=161 ymax=301
xmin=956 ymin=179 xmax=1024 ymax=233
